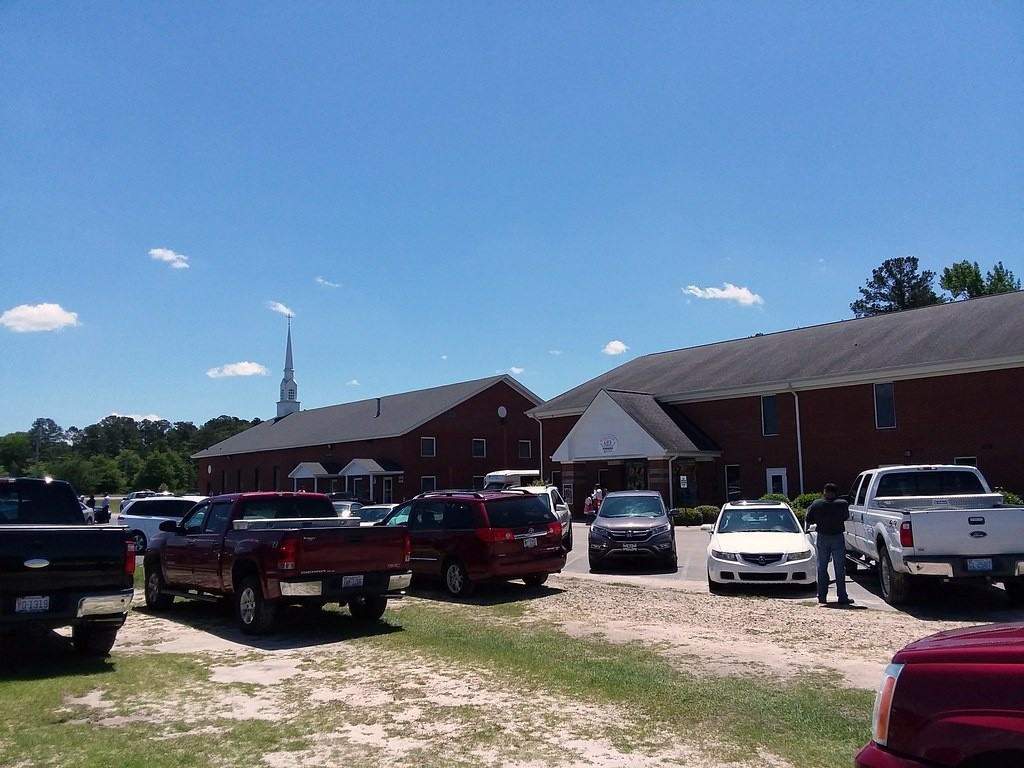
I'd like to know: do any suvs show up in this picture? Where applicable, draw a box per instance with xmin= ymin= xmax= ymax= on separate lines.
xmin=587 ymin=489 xmax=682 ymax=572
xmin=114 ymin=488 xmax=269 ymax=555
xmin=503 ymin=483 xmax=574 ymax=553
xmin=371 ymin=485 xmax=569 ymax=600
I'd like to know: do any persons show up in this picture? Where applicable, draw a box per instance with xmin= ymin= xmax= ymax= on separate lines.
xmin=78 ymin=495 xmax=85 ymax=502
xmin=584 ymin=483 xmax=602 ymax=525
xmin=102 ymin=492 xmax=110 ymax=523
xmin=805 ymin=482 xmax=854 ymax=605
xmin=86 ymin=494 xmax=96 ymax=511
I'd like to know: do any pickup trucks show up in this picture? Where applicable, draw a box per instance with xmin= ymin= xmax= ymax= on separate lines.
xmin=837 ymin=464 xmax=1024 ymax=608
xmin=142 ymin=491 xmax=411 ymax=637
xmin=0 ymin=475 xmax=136 ymax=660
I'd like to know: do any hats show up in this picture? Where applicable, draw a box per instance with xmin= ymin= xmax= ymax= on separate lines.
xmin=823 ymin=483 xmax=836 ymax=493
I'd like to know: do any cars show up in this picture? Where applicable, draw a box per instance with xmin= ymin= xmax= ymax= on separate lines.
xmin=325 ymin=469 xmax=548 ymax=527
xmin=848 ymin=617 xmax=1024 ymax=768
xmin=93 ymin=505 xmax=112 ymax=521
xmin=700 ymin=501 xmax=821 ymax=592
xmin=78 ymin=501 xmax=96 ymax=525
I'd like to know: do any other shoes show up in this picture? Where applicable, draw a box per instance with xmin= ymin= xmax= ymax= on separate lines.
xmin=840 ymin=598 xmax=854 ymax=604
xmin=818 ymin=599 xmax=826 ymax=603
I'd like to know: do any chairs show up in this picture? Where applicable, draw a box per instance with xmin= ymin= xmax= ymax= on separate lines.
xmin=418 ymin=512 xmax=441 ymax=530
